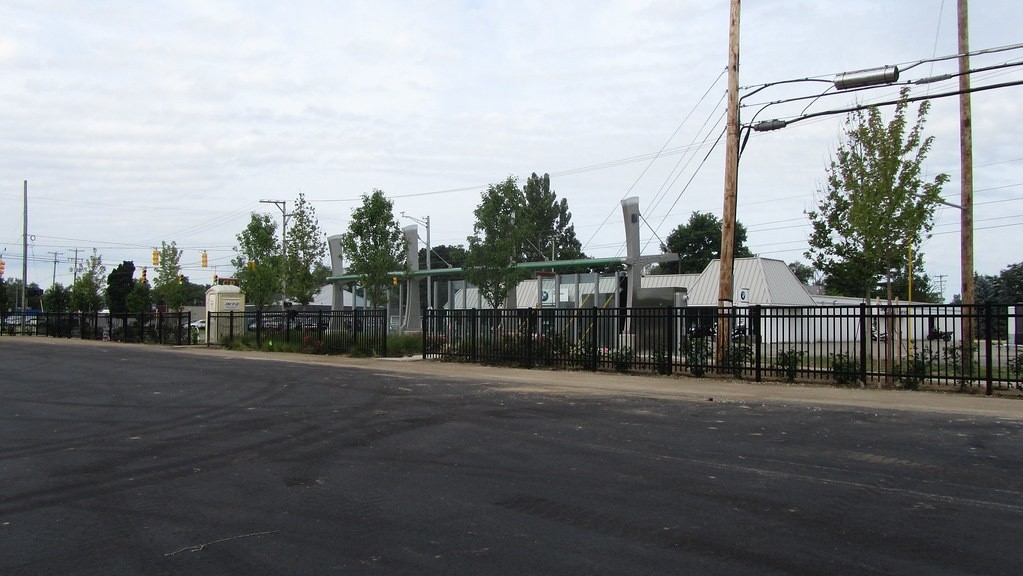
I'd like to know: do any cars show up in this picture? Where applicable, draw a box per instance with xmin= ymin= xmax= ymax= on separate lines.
xmin=183 ymin=318 xmax=207 ymax=329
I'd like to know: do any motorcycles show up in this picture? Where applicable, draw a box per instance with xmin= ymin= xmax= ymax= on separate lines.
xmin=711 ymin=322 xmax=745 ymax=342
xmin=871 ymin=327 xmax=890 ymax=343
xmin=927 ymin=326 xmax=954 ymax=341
